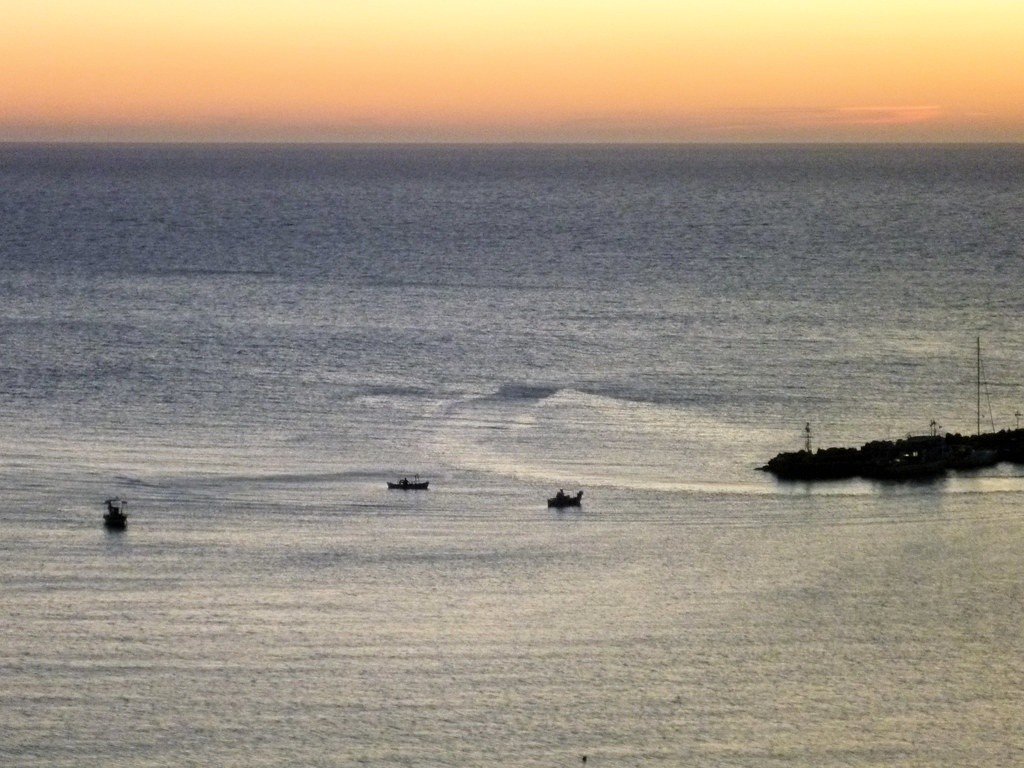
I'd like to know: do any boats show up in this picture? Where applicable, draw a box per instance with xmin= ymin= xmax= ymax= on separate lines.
xmin=548 ymin=490 xmax=584 ymax=508
xmin=103 ymin=496 xmax=127 ymax=529
xmin=388 ymin=479 xmax=429 ymax=490
